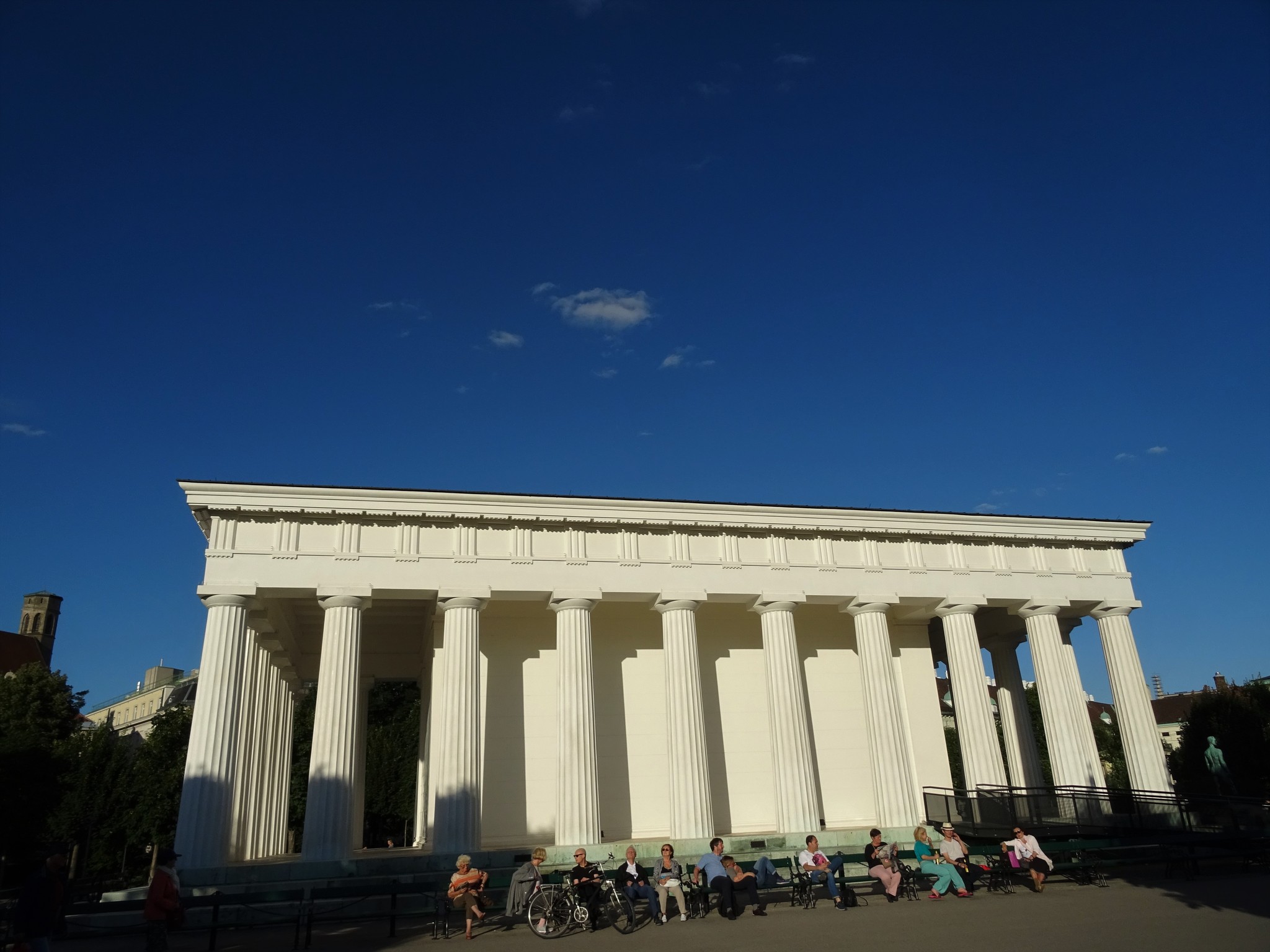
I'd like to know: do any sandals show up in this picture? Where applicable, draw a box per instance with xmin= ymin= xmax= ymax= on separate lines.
xmin=466 ymin=933 xmax=472 ymax=940
xmin=478 ymin=912 xmax=486 ymax=920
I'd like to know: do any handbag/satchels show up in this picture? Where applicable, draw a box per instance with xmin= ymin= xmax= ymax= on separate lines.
xmin=1008 ymin=851 xmax=1020 ymax=868
xmin=881 ymin=858 xmax=892 ymax=868
xmin=842 ymin=888 xmax=868 ymax=907
xmin=812 ymin=853 xmax=827 ymax=866
xmin=659 ymin=878 xmax=681 ymax=887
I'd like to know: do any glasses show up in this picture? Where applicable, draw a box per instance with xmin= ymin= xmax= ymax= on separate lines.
xmin=573 ymin=853 xmax=584 ymax=858
xmin=1014 ymin=830 xmax=1021 ymax=835
xmin=662 ymin=848 xmax=671 ymax=851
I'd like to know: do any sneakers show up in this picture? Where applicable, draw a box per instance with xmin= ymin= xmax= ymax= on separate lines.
xmin=680 ymin=914 xmax=687 ymax=921
xmin=653 ymin=918 xmax=662 ymax=925
xmin=817 ymin=871 xmax=827 ymax=881
xmin=628 ymin=921 xmax=635 ymax=927
xmin=727 ymin=909 xmax=736 ymax=920
xmin=661 ymin=915 xmax=667 ymax=922
xmin=752 ymin=908 xmax=767 ymax=916
xmin=776 ymin=877 xmax=790 ymax=884
xmin=835 ymin=900 xmax=846 ymax=910
xmin=536 ymin=924 xmax=553 ymax=932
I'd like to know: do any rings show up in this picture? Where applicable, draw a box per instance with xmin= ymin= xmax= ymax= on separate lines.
xmin=962 ymin=866 xmax=964 ymax=868
xmin=1001 ymin=848 xmax=1003 ymax=850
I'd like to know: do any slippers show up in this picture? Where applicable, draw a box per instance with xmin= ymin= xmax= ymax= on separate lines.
xmin=928 ymin=894 xmax=942 ymax=899
xmin=958 ymin=890 xmax=973 ymax=896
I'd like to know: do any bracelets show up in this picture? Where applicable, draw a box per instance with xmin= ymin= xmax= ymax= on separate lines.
xmin=957 ymin=862 xmax=961 ymax=866
xmin=1030 ymin=855 xmax=1034 ymax=858
xmin=481 ymin=883 xmax=484 ymax=885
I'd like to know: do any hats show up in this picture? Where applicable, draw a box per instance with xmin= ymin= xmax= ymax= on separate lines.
xmin=940 ymin=822 xmax=955 ymax=830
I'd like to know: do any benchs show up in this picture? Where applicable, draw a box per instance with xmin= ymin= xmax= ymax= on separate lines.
xmin=443 ymin=835 xmax=1270 ymax=932
xmin=304 ymin=881 xmax=441 ymax=951
xmin=54 ymin=883 xmax=304 ymax=952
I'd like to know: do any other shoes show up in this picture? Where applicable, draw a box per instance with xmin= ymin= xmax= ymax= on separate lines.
xmin=1034 ymin=878 xmax=1040 ymax=891
xmin=893 ymin=896 xmax=899 ymax=901
xmin=886 ymin=893 xmax=893 ymax=903
xmin=1038 ymin=883 xmax=1045 ymax=892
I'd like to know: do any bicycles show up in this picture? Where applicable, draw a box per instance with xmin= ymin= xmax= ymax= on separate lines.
xmin=526 ymin=852 xmax=636 ymax=940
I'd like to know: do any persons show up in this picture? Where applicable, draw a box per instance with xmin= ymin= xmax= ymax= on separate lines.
xmin=798 ymin=835 xmax=848 ymax=911
xmin=447 ymin=854 xmax=489 ymax=940
xmin=142 ymin=848 xmax=187 ymax=952
xmin=999 ymin=826 xmax=1054 ymax=893
xmin=865 ymin=828 xmax=901 ymax=903
xmin=1204 ymin=736 xmax=1239 ymax=803
xmin=612 ymin=846 xmax=663 ymax=935
xmin=653 ymin=843 xmax=688 ymax=922
xmin=569 ymin=848 xmax=614 ymax=932
xmin=387 ymin=840 xmax=394 ymax=848
xmin=505 ymin=847 xmax=562 ymax=933
xmin=913 ymin=826 xmax=973 ymax=898
xmin=692 ymin=838 xmax=791 ymax=920
xmin=940 ymin=822 xmax=985 ymax=892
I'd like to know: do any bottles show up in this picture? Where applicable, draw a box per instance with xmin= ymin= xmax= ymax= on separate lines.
xmin=963 ymin=858 xmax=970 ymax=874
xmin=935 ymin=850 xmax=940 ymax=865
